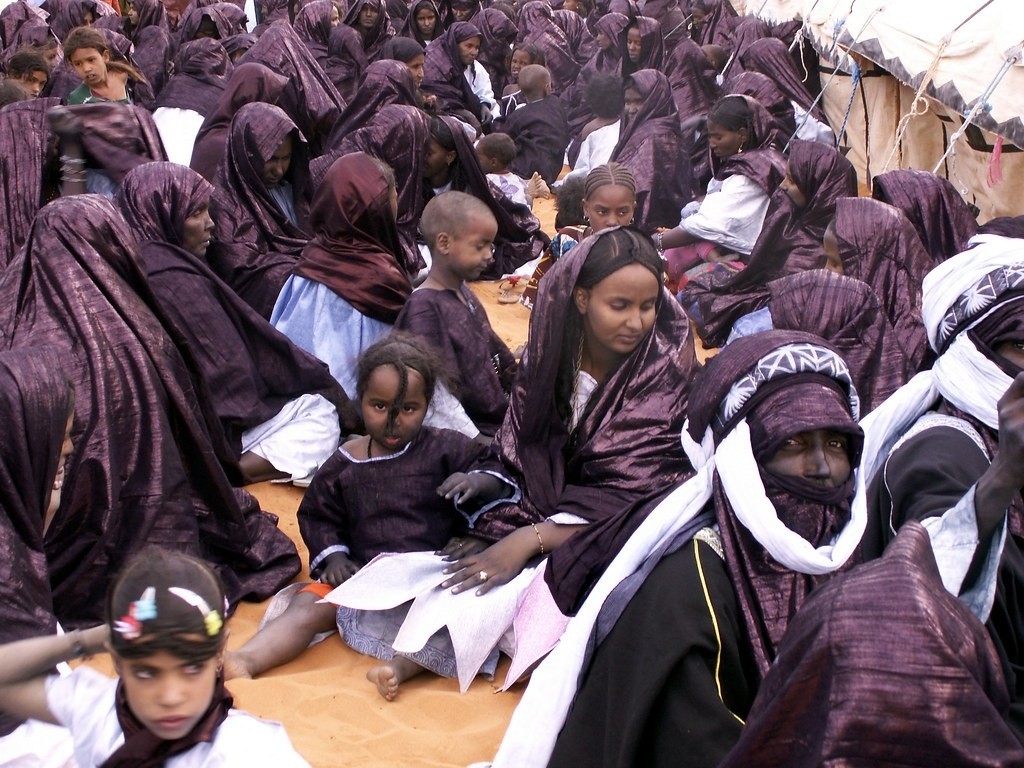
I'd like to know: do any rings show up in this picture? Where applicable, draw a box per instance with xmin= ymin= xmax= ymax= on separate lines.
xmin=459 ymin=542 xmax=463 ymax=548
xmin=480 ymin=570 xmax=488 ymax=582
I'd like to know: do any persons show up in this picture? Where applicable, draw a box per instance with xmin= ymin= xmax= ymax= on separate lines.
xmin=722 ymin=521 xmax=1023 ymax=768
xmin=435 ymin=225 xmax=702 ymax=669
xmin=471 ymin=328 xmax=867 ymax=768
xmin=0 ymin=0 xmax=1024 ymax=639
xmin=859 ymin=237 xmax=1023 ymax=702
xmin=0 ymin=546 xmax=309 ymax=768
xmin=222 ymin=335 xmax=522 ymax=700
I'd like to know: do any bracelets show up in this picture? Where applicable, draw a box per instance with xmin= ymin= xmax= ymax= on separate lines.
xmin=659 ymin=233 xmax=663 ymax=251
xmin=72 ymin=631 xmax=93 ymax=662
xmin=532 ymin=523 xmax=544 ymax=553
